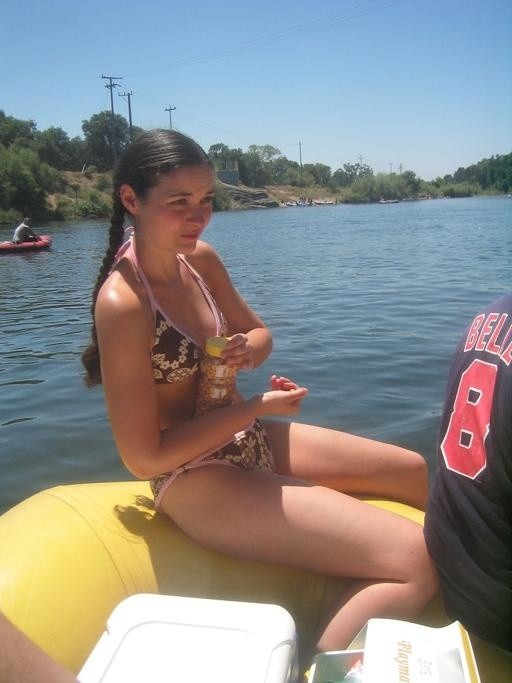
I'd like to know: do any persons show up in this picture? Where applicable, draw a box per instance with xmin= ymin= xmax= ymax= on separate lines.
xmin=1 ymin=609 xmax=82 ymax=682
xmin=14 ymin=217 xmax=40 ymax=241
xmin=81 ymin=127 xmax=443 ymax=653
xmin=421 ymin=274 xmax=511 ymax=655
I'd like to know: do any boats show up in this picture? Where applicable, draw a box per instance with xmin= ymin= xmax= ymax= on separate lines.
xmin=286 ymin=202 xmax=335 ymax=206
xmin=378 ymin=199 xmax=398 ymax=204
xmin=0 ymin=234 xmax=52 ymax=251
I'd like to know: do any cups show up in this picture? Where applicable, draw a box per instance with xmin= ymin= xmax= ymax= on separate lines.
xmin=194 ymin=336 xmax=236 ymax=417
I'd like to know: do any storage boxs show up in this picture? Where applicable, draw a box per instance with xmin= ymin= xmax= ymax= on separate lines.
xmin=76 ymin=593 xmax=300 ymax=683
